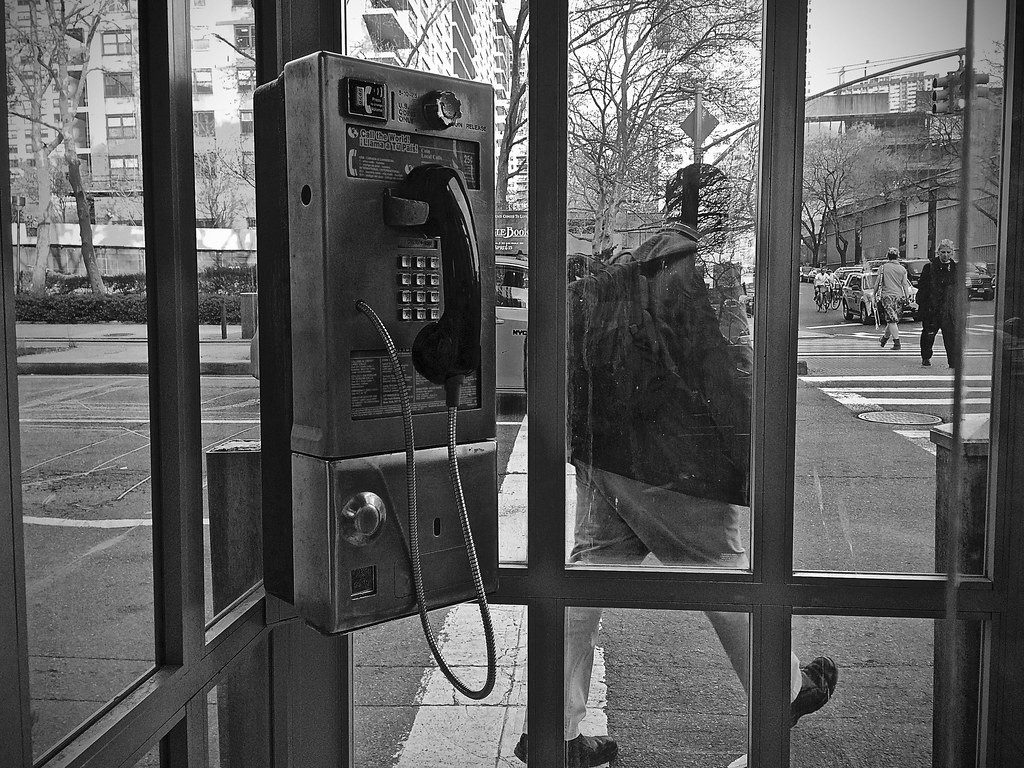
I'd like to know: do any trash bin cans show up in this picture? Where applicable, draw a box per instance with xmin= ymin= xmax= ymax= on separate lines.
xmin=927 ymin=413 xmax=989 ymax=768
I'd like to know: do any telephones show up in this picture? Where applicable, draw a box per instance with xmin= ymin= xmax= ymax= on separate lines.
xmin=251 ymin=48 xmax=496 ymax=633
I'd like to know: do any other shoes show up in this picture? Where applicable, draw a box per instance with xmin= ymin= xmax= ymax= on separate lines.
xmin=949 ymin=364 xmax=955 ymax=368
xmin=922 ymin=359 xmax=931 ymax=366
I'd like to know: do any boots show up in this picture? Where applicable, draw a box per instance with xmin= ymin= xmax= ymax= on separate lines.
xmin=879 ymin=336 xmax=889 ymax=347
xmin=891 ymin=339 xmax=901 ymax=350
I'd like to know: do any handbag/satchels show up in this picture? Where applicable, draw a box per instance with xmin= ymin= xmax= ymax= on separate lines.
xmin=916 ymin=262 xmax=936 ymax=309
xmin=876 ymin=265 xmax=884 ymax=303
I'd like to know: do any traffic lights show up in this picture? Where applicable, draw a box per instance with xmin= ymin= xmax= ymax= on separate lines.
xmin=932 ymin=71 xmax=956 ymax=116
xmin=973 ymin=69 xmax=990 ymax=111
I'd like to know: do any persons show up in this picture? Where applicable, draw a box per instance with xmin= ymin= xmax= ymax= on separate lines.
xmin=873 ymin=246 xmax=910 ymax=350
xmin=813 ymin=268 xmax=833 ymax=301
xmin=916 ymin=239 xmax=964 ymax=368
xmin=825 ymin=268 xmax=840 ymax=302
xmin=515 ymin=164 xmax=838 ymax=768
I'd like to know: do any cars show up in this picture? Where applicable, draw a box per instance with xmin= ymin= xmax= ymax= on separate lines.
xmin=831 ymin=258 xmax=932 ymax=325
xmin=494 ymin=254 xmax=580 ymax=395
xmin=799 ymin=267 xmax=833 ymax=283
xmin=956 ymin=260 xmax=995 ymax=302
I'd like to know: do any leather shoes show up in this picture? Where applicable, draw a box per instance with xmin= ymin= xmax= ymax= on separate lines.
xmin=790 ymin=656 xmax=839 ymax=728
xmin=513 ymin=733 xmax=618 ymax=768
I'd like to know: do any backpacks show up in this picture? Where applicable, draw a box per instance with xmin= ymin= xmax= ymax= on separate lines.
xmin=567 ymin=241 xmax=704 ymax=432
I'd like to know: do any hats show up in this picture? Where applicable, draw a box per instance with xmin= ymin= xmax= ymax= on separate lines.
xmin=938 ymin=239 xmax=955 ymax=256
xmin=888 ymin=248 xmax=898 ymax=255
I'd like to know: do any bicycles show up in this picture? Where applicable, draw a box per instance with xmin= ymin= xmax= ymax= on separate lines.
xmin=813 ymin=282 xmax=843 ymax=313
